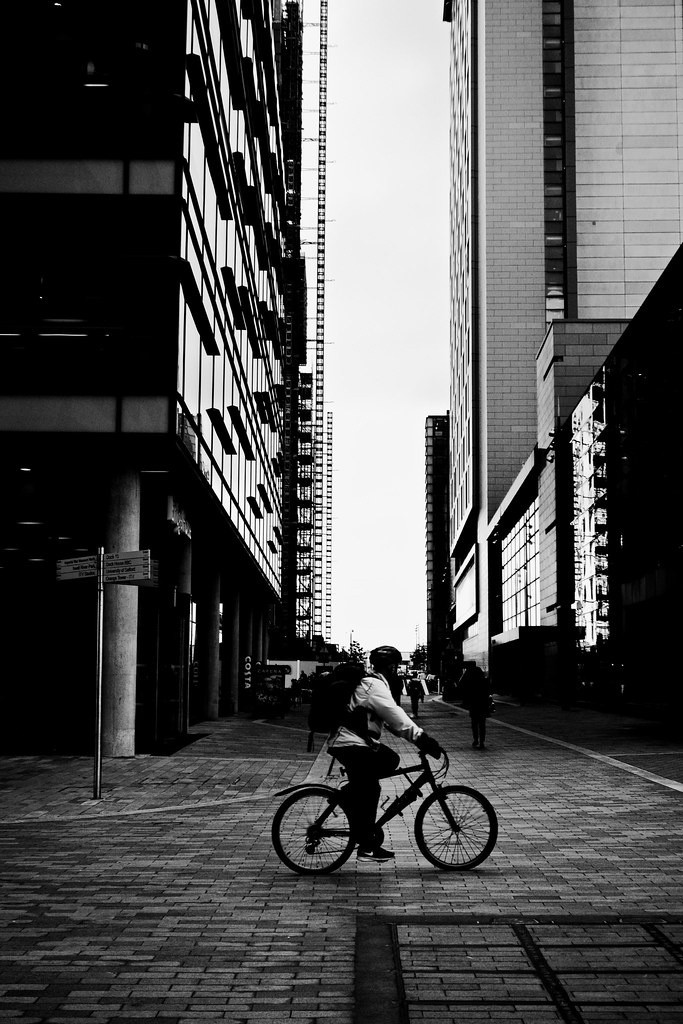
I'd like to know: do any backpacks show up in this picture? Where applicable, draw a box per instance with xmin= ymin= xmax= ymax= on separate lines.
xmin=307 ymin=664 xmax=381 ymax=752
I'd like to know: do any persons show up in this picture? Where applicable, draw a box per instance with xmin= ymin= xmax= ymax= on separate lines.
xmin=407 ymin=671 xmax=425 ymax=719
xmin=328 ymin=646 xmax=441 ymax=862
xmin=464 ymin=667 xmax=492 ymax=750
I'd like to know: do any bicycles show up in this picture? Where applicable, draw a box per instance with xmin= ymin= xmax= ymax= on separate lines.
xmin=271 ymin=734 xmax=500 ymax=877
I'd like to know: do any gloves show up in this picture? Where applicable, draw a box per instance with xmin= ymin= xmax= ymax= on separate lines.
xmin=414 ymin=732 xmax=441 ymax=760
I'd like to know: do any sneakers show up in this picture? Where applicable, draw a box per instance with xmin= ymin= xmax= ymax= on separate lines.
xmin=356 ymin=844 xmax=395 ymax=862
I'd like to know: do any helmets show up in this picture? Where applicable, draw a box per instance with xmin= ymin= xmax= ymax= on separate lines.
xmin=369 ymin=646 xmax=402 ymax=665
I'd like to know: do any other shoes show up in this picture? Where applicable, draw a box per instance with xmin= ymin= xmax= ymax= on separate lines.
xmin=472 ymin=740 xmax=478 ymax=747
xmin=480 ymin=742 xmax=484 ymax=748
